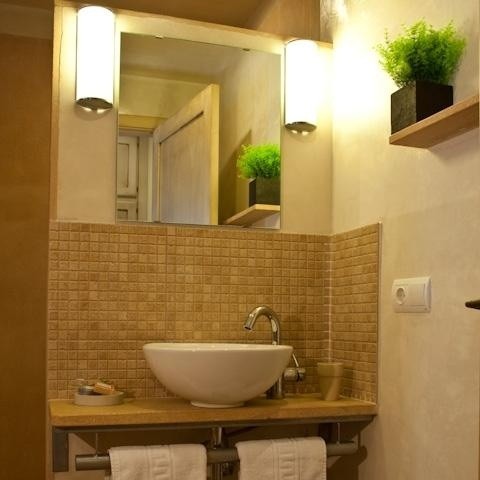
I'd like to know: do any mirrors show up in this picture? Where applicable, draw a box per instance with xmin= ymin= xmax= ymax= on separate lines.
xmin=113 ymin=30 xmax=284 ymax=233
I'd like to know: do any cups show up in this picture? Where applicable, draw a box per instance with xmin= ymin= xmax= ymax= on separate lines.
xmin=317 ymin=362 xmax=345 ymax=402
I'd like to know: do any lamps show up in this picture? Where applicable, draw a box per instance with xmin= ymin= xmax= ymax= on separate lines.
xmin=73 ymin=2 xmax=119 ymax=114
xmin=283 ymin=38 xmax=322 ymax=134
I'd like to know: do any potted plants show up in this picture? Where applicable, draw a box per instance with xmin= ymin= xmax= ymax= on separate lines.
xmin=375 ymin=15 xmax=469 ymax=137
xmin=233 ymin=141 xmax=281 ymax=205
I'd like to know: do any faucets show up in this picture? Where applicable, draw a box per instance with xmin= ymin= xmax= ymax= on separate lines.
xmin=244 ymin=305 xmax=282 ymax=398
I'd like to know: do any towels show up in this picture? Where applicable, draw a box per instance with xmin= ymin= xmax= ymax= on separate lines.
xmin=105 ymin=435 xmax=328 ymax=480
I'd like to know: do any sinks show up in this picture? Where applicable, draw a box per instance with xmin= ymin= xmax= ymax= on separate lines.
xmin=142 ymin=341 xmax=293 ymax=408
